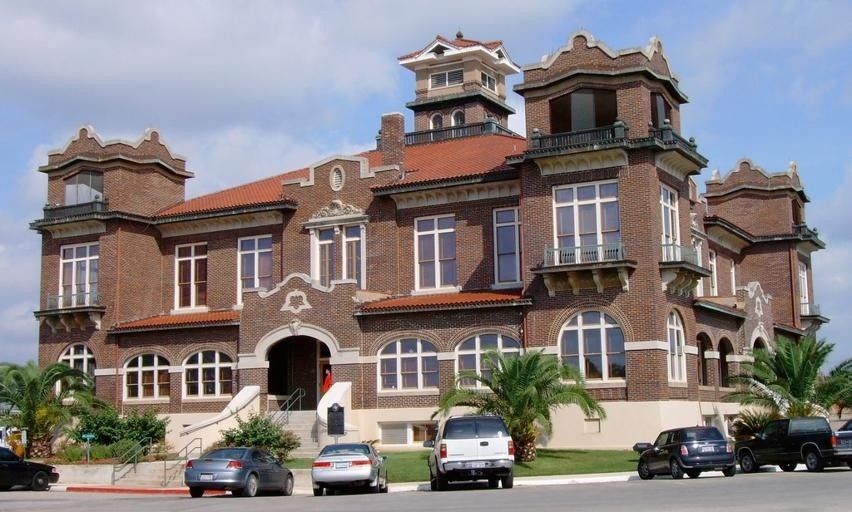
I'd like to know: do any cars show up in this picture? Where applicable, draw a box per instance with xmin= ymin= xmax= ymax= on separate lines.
xmin=185 ymin=447 xmax=293 ymax=497
xmin=311 ymin=442 xmax=388 ymax=496
xmin=0 ymin=446 xmax=59 ymax=491
xmin=423 ymin=415 xmax=516 ymax=491
xmin=638 ymin=425 xmax=736 ymax=479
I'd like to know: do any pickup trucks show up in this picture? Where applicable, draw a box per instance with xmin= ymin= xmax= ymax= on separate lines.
xmin=736 ymin=416 xmax=852 ymax=472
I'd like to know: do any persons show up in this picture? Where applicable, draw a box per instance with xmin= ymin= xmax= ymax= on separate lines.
xmin=323 ymin=366 xmax=333 ymax=394
